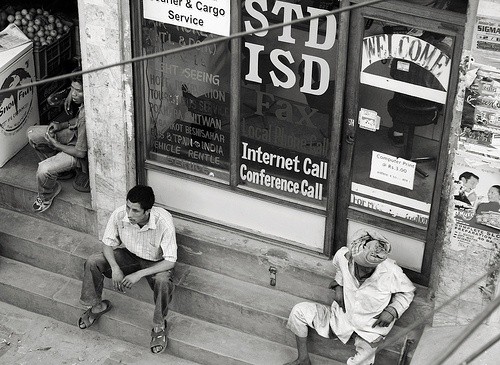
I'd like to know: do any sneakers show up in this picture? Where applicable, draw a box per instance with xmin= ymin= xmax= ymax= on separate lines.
xmin=57 ymin=169 xmax=74 ymax=180
xmin=32 ymin=179 xmax=62 ymax=214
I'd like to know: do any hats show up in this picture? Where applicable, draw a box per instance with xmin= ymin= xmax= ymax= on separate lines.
xmin=344 ymin=226 xmax=392 ymax=268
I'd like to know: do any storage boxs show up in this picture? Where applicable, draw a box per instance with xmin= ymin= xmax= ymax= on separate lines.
xmin=34 ymin=25 xmax=79 ymax=126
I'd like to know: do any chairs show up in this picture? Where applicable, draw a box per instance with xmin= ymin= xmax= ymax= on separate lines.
xmin=183 ymin=85 xmax=230 ymax=141
xmin=226 ymin=37 xmax=288 ymax=129
xmin=390 ymin=93 xmax=439 ymax=176
xmin=298 ymin=57 xmax=335 ymax=147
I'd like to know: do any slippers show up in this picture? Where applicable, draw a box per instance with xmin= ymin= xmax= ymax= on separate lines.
xmin=149 ymin=319 xmax=168 ymax=355
xmin=78 ymin=299 xmax=112 ymax=329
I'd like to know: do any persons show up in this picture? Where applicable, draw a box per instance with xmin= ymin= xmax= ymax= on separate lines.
xmin=284 ymin=228 xmax=416 ymax=365
xmin=77 ymin=185 xmax=178 ymax=355
xmin=64 ymin=89 xmax=74 ymax=116
xmin=26 ymin=75 xmax=89 ymax=214
xmin=454 ymin=171 xmax=500 ymax=235
